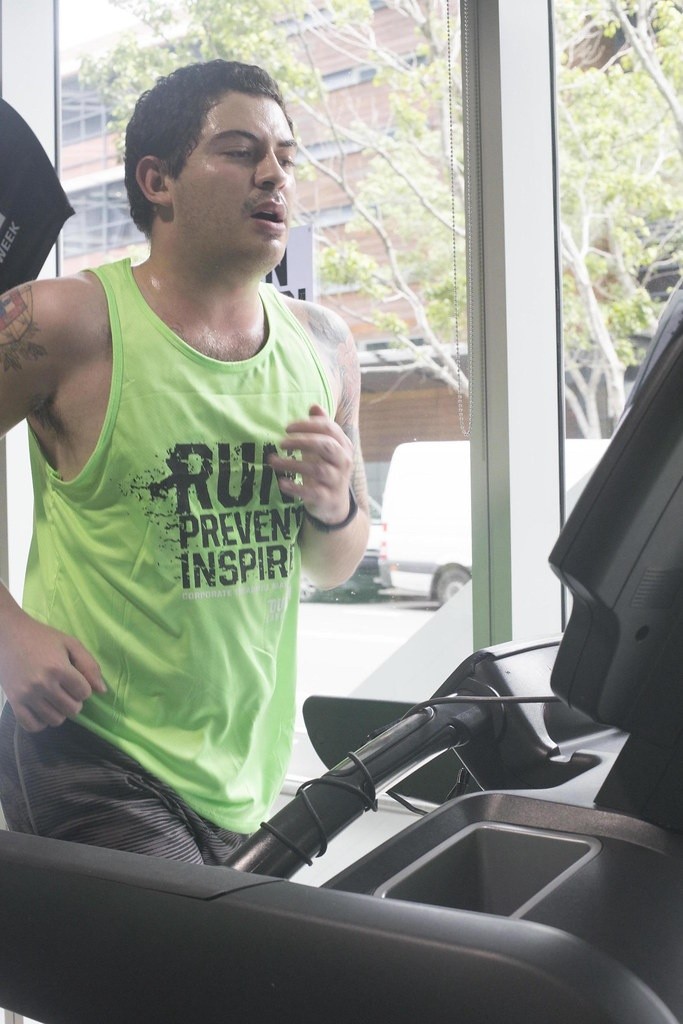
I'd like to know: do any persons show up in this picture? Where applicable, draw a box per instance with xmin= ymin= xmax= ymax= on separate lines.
xmin=2 ymin=56 xmax=370 ymax=871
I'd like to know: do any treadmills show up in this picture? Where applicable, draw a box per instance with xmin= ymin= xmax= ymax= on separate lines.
xmin=1 ymin=267 xmax=683 ymax=1024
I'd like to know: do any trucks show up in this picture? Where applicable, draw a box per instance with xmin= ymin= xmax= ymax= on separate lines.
xmin=379 ymin=438 xmax=610 ymax=611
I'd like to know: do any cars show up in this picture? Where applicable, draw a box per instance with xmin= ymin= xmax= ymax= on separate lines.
xmin=287 ymin=492 xmax=387 ymax=599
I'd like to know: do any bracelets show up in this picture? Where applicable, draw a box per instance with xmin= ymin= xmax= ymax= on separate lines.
xmin=293 ymin=486 xmax=361 ymax=531
